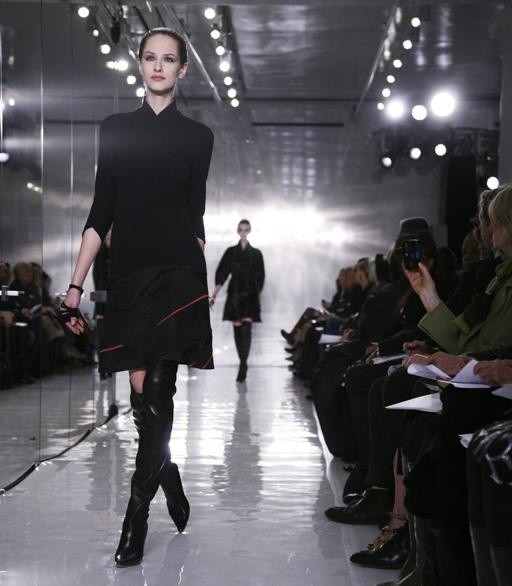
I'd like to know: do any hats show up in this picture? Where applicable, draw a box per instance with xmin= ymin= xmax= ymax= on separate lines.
xmin=388 ymin=217 xmax=436 ymax=261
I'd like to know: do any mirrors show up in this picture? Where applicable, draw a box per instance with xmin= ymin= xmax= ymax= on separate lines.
xmin=0 ymin=0 xmax=133 ymax=497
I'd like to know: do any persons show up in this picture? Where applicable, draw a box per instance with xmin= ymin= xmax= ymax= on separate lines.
xmin=283 ymin=187 xmax=512 ymax=586
xmin=209 ymin=217 xmax=268 ymax=386
xmin=0 ymin=256 xmax=99 ymax=393
xmin=58 ymin=25 xmax=216 ymax=567
xmin=92 ymin=226 xmax=114 ymax=381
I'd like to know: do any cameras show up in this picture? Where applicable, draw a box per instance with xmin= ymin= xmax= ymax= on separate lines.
xmin=403 ymin=239 xmax=419 ymax=272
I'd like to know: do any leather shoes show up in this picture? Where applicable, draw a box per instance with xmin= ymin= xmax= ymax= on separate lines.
xmin=285 ymin=347 xmax=296 ymax=353
xmin=282 ymin=330 xmax=294 ymax=344
xmin=304 ymin=382 xmax=312 ymax=387
xmin=342 ymin=489 xmax=363 ymax=504
xmin=325 ymin=487 xmax=392 ymax=523
xmin=293 ymin=372 xmax=307 ymax=380
xmin=286 ymin=355 xmax=295 ymax=361
xmin=305 ymin=394 xmax=314 ymax=401
xmin=351 ymin=524 xmax=409 ymax=569
xmin=288 ymin=364 xmax=295 ymax=368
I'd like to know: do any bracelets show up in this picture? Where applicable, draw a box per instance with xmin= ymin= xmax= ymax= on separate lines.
xmin=211 ymin=295 xmax=216 ymax=299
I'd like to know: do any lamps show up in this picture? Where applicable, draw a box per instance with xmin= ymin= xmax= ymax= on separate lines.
xmin=64 ymin=3 xmax=147 ymax=104
xmin=372 ymin=12 xmax=423 ymax=112
xmin=370 ymin=123 xmax=448 ymax=181
xmin=202 ymin=1 xmax=248 ymax=112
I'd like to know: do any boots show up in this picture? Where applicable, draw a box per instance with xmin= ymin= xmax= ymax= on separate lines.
xmin=131 ymin=380 xmax=189 ymax=534
xmin=234 ymin=321 xmax=250 ymax=382
xmin=114 ymin=396 xmax=176 ymax=566
